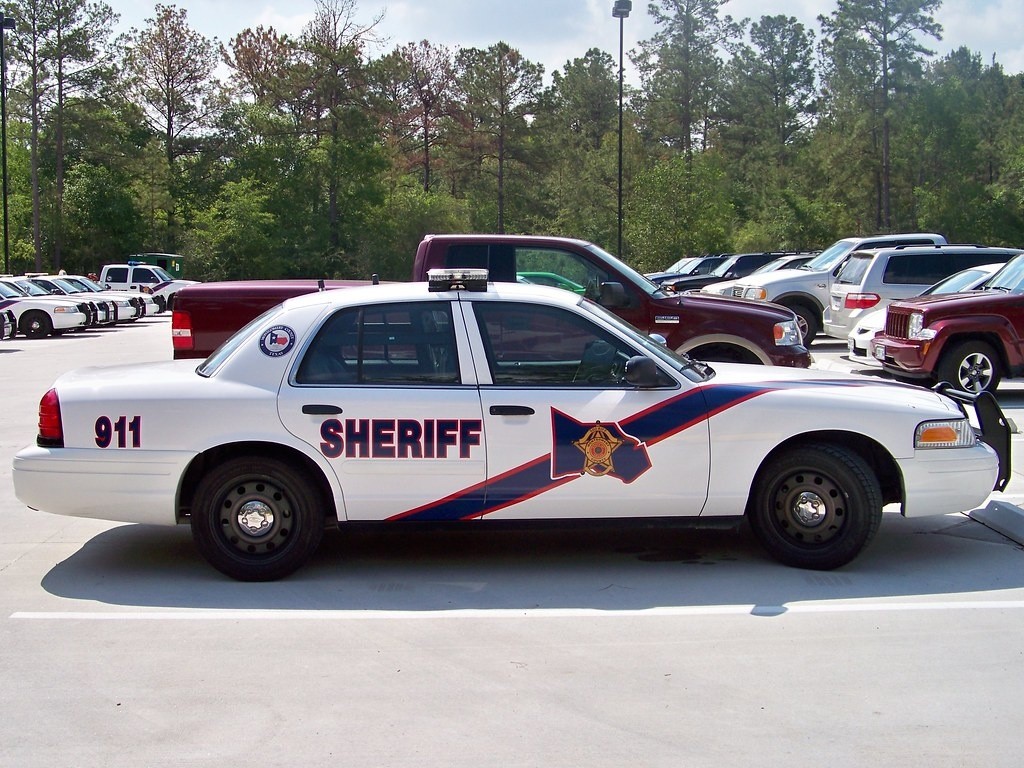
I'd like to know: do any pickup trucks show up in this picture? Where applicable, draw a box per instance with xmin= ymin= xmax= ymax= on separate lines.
xmin=170 ymin=231 xmax=813 ymax=375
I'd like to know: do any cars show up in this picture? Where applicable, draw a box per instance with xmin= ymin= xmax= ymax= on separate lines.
xmin=14 ymin=266 xmax=1024 ymax=585
xmin=0 ymin=263 xmax=202 ymax=345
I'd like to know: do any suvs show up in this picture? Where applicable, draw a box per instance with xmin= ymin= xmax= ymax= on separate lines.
xmin=510 ymin=233 xmax=1024 ymax=405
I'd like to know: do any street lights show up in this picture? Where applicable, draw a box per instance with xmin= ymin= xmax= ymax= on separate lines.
xmin=612 ymin=0 xmax=632 ymax=261
